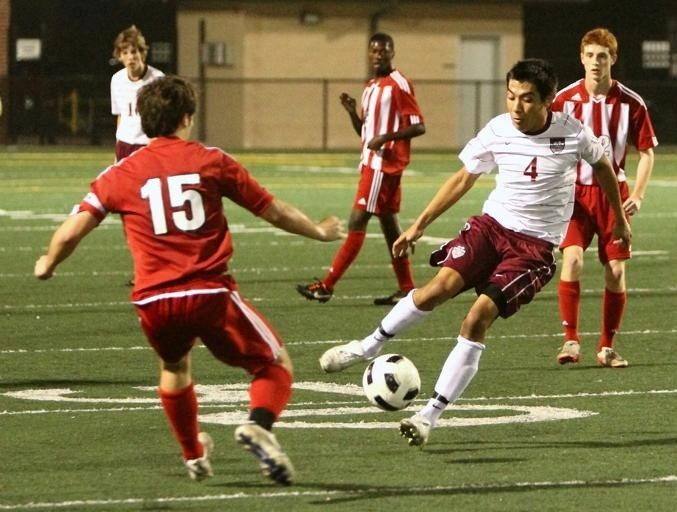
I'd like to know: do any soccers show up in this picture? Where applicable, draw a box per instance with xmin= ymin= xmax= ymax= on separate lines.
xmin=362 ymin=354 xmax=420 ymax=411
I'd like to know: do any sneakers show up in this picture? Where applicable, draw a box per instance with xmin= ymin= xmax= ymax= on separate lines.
xmin=595 ymin=346 xmax=628 ymax=368
xmin=318 ymin=338 xmax=384 ymax=374
xmin=182 ymin=430 xmax=214 ymax=481
xmin=128 ymin=272 xmax=134 ymax=287
xmin=233 ymin=420 xmax=296 ymax=486
xmin=296 ymin=278 xmax=336 ymax=303
xmin=558 ymin=340 xmax=581 ymax=364
xmin=373 ymin=291 xmax=408 ymax=306
xmin=400 ymin=411 xmax=431 ymax=447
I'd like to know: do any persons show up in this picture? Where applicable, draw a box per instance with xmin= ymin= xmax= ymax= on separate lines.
xmin=34 ymin=73 xmax=350 ymax=486
xmin=319 ymin=59 xmax=631 ymax=450
xmin=295 ymin=34 xmax=426 ymax=305
xmin=549 ymin=28 xmax=658 ymax=367
xmin=111 ymin=25 xmax=167 ymax=285
